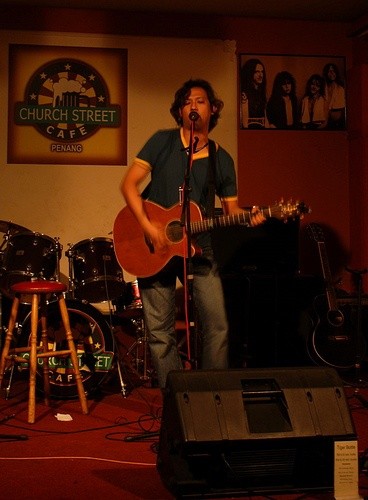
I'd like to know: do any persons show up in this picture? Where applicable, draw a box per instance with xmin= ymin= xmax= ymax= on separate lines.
xmin=121 ymin=78 xmax=267 ymax=388
xmin=241 ymin=59 xmax=345 ymax=131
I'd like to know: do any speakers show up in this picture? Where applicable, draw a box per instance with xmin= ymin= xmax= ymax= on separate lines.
xmin=157 ymin=367 xmax=357 ymax=500
xmin=208 ymin=207 xmax=302 ymax=368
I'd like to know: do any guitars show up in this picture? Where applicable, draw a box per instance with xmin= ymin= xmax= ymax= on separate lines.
xmin=309 ymin=229 xmax=364 ymax=370
xmin=112 ymin=199 xmax=314 ymax=279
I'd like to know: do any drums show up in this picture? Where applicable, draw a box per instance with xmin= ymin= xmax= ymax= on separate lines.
xmin=115 ymin=280 xmax=144 ymax=319
xmin=66 ymin=236 xmax=127 ymax=303
xmin=16 ymin=298 xmax=116 ymax=400
xmin=1 ymin=231 xmax=63 ymax=286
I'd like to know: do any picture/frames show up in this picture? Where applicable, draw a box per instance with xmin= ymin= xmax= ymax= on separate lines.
xmin=238 ymin=53 xmax=348 ymax=130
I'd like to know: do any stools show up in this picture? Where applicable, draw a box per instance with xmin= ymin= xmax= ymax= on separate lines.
xmin=0 ymin=281 xmax=89 ymax=423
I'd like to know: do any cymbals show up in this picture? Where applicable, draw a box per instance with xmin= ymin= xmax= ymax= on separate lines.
xmin=0 ymin=220 xmax=33 ymax=235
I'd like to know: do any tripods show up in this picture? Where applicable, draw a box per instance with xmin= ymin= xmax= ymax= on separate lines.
xmin=94 ymin=315 xmax=196 ymax=400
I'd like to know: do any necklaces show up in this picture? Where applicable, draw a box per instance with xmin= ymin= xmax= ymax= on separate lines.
xmin=195 ymin=143 xmax=209 ymax=153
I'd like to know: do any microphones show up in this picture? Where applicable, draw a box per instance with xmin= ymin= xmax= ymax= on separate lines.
xmin=189 ymin=111 xmax=199 ymax=120
